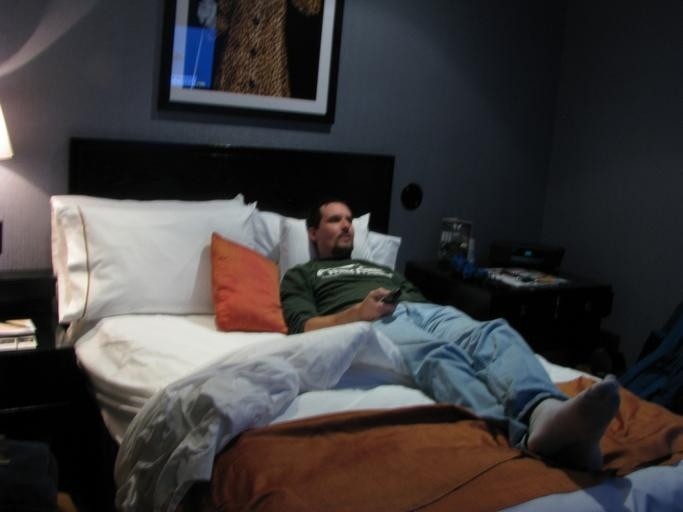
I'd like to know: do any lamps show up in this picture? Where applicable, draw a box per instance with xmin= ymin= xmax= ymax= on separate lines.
xmin=0 ymin=105 xmax=14 ymax=160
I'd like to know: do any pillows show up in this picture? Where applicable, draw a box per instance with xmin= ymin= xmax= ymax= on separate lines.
xmin=55 ymin=201 xmax=258 ymax=325
xmin=208 ymin=228 xmax=289 ymax=333
xmin=277 ymin=211 xmax=374 ymax=281
xmin=49 ymin=193 xmax=248 ymax=282
xmin=258 ymin=210 xmax=402 ymax=272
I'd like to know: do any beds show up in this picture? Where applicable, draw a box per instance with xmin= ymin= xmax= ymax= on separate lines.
xmin=69 ymin=134 xmax=683 ymax=512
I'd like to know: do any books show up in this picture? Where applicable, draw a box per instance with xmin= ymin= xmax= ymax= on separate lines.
xmin=478 ymin=264 xmax=568 ymax=290
xmin=0 ymin=318 xmax=39 ymax=351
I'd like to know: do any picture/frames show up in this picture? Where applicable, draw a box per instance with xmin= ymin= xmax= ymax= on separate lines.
xmin=158 ymin=0 xmax=344 ymax=126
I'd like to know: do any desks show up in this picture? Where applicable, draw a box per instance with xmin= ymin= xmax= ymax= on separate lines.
xmin=404 ymin=260 xmax=613 ymax=353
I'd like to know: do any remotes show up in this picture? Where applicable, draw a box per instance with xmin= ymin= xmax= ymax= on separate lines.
xmin=380 ymin=288 xmax=401 ymax=304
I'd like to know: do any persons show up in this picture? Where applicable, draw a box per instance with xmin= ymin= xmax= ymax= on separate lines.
xmin=278 ymin=196 xmax=622 ymax=473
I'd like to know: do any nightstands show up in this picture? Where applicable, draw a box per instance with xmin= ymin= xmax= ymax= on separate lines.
xmin=0 ymin=333 xmax=68 ymax=449
